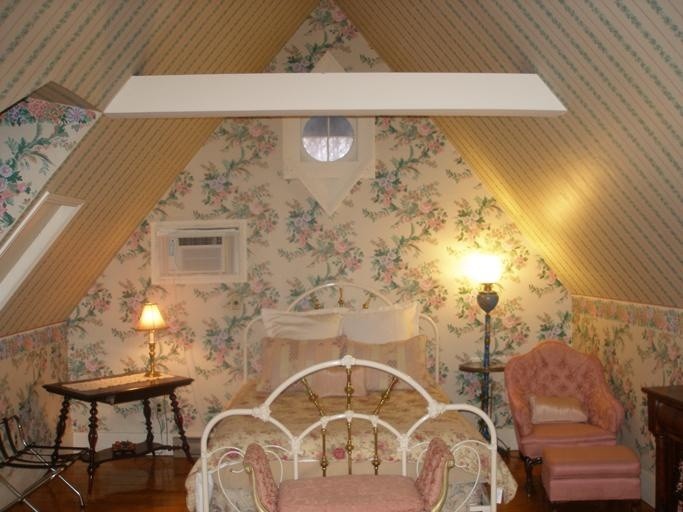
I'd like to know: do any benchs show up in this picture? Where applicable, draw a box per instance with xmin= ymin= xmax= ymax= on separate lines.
xmin=243 ymin=436 xmax=454 ymax=512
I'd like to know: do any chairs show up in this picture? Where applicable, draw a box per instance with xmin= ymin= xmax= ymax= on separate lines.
xmin=504 ymin=341 xmax=641 ymax=498
xmin=0 ymin=415 xmax=89 ymax=512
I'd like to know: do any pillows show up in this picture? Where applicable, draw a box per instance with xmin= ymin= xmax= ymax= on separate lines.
xmin=529 ymin=395 xmax=589 ymax=425
xmin=255 ymin=302 xmax=429 ymax=397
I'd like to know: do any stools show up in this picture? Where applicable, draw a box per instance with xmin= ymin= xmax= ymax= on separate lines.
xmin=541 ymin=446 xmax=642 ymax=512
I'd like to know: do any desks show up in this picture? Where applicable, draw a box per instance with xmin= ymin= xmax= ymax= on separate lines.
xmin=641 ymin=385 xmax=683 ymax=512
xmin=42 ymin=371 xmax=194 ymax=495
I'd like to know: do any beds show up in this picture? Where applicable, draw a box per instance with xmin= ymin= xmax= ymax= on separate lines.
xmin=183 ymin=283 xmax=518 ymax=512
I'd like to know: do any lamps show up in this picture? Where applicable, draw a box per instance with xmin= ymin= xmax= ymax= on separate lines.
xmin=459 ymin=255 xmax=508 ymax=456
xmin=136 ymin=303 xmax=169 ymax=377
